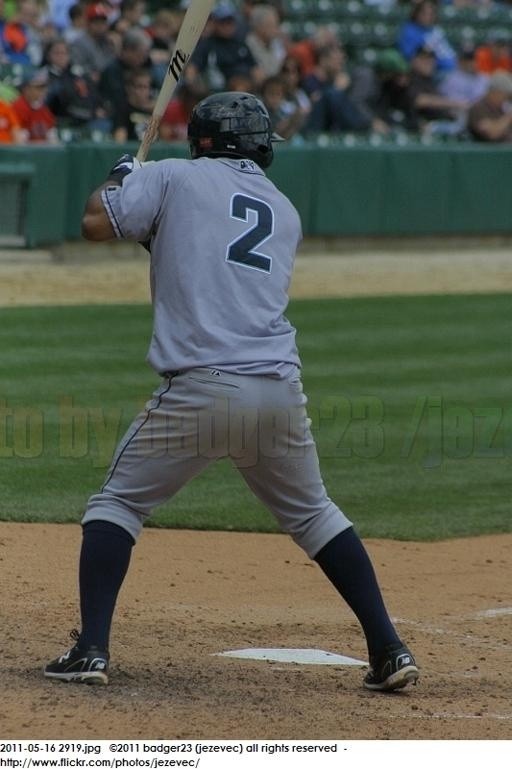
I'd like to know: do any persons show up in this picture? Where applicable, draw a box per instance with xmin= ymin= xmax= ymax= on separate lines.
xmin=42 ymin=93 xmax=418 ymax=692
xmin=0 ymin=0 xmax=512 ymax=151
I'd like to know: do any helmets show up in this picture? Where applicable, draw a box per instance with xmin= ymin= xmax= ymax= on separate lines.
xmin=188 ymin=91 xmax=286 ymax=168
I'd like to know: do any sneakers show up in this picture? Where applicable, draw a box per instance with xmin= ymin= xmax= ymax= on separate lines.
xmin=363 ymin=646 xmax=419 ymax=689
xmin=44 ymin=629 xmax=109 ymax=685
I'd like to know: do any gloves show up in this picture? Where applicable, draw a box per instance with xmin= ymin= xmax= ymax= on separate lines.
xmin=106 ymin=154 xmax=156 ymax=187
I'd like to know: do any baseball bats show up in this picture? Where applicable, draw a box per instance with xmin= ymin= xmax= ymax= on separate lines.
xmin=136 ymin=1 xmax=211 ymax=164
